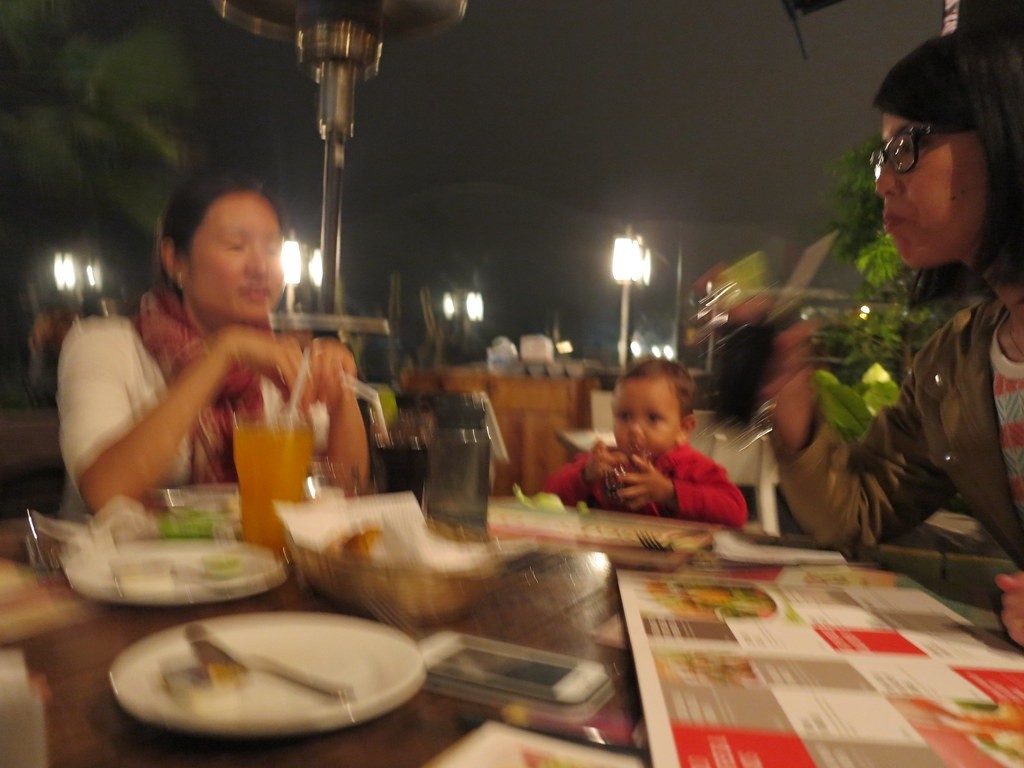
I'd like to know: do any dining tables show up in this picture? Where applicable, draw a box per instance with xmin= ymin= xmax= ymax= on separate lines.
xmin=2 ymin=489 xmax=1024 ymax=767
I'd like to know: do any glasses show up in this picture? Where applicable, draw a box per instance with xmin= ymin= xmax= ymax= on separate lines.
xmin=869 ymin=124 xmax=975 ymax=175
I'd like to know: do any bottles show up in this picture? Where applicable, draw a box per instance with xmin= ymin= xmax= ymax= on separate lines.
xmin=422 ymin=390 xmax=493 ymax=540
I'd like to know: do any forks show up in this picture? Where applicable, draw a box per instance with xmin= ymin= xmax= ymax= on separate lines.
xmin=635 ymin=528 xmax=710 ymax=550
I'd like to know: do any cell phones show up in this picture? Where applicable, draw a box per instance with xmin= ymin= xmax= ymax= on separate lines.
xmin=417 ymin=631 xmax=611 ymax=713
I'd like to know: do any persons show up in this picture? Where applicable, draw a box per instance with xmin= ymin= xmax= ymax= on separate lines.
xmin=56 ymin=175 xmax=369 ymax=510
xmin=682 ymin=26 xmax=1024 ymax=655
xmin=546 ymin=360 xmax=747 ymax=528
xmin=29 ymin=306 xmax=75 ymax=407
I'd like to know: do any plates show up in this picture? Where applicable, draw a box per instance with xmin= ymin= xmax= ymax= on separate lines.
xmin=104 ymin=609 xmax=427 ymax=737
xmin=66 ymin=538 xmax=287 ymax=606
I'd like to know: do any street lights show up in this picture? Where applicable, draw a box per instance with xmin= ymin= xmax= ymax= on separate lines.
xmin=611 ymin=224 xmax=653 ymax=372
xmin=214 ymin=0 xmax=470 ymax=343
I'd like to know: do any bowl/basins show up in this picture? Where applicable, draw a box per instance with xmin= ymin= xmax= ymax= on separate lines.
xmin=641 ymin=578 xmax=786 ymax=629
xmin=280 ymin=515 xmax=502 ymax=625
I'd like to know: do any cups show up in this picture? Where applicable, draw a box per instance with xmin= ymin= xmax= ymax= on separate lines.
xmin=307 ymin=458 xmax=359 ymax=501
xmin=229 ymin=406 xmax=313 ymax=560
xmin=700 ymin=320 xmax=778 ymax=432
xmin=601 ymin=447 xmax=652 ymax=501
